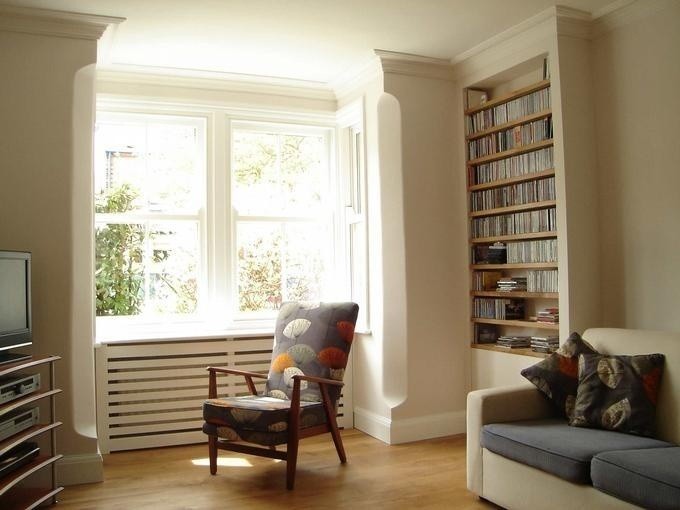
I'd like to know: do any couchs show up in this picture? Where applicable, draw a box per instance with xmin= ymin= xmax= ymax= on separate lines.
xmin=468 ymin=327 xmax=678 ymax=509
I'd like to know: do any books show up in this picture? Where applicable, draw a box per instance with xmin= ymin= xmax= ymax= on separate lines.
xmin=464 ymin=86 xmax=559 ymax=353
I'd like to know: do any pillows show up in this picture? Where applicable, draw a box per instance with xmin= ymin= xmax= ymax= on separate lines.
xmin=521 ymin=333 xmax=665 ymax=440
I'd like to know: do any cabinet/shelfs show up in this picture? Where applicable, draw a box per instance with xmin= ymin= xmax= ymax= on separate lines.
xmin=464 ymin=54 xmax=566 ymax=356
xmin=1 ymin=355 xmax=65 ymax=510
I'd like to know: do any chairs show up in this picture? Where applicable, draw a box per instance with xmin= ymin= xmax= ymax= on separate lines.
xmin=201 ymin=300 xmax=361 ymax=491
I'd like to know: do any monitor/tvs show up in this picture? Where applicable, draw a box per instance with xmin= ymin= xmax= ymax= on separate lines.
xmin=0 ymin=250 xmax=33 ymax=365
